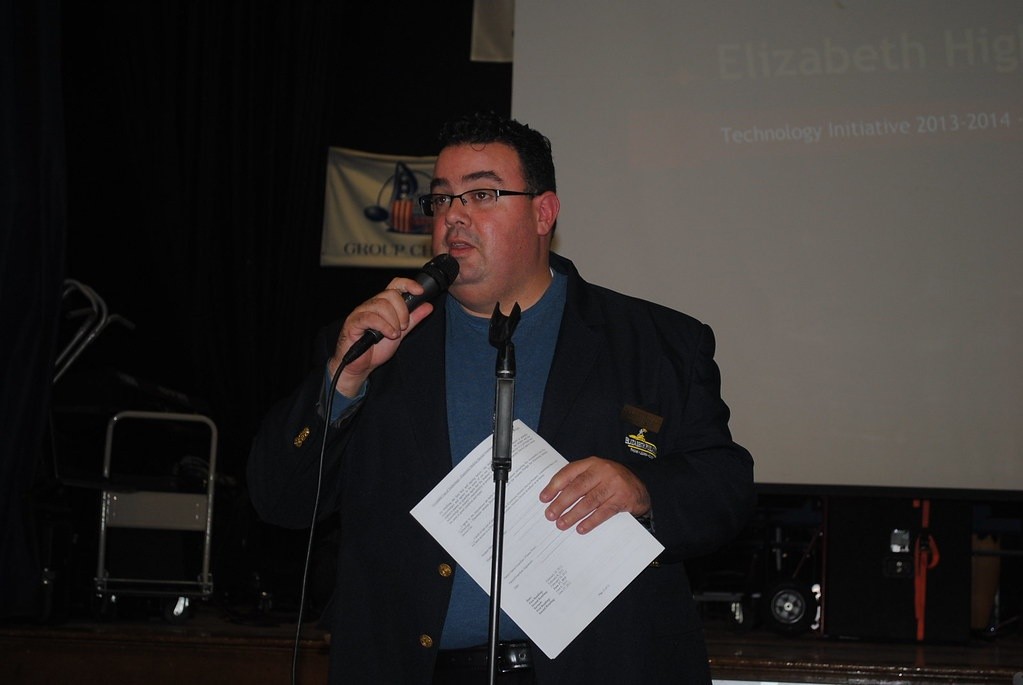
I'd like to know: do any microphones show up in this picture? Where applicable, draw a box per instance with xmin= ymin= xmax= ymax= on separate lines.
xmin=342 ymin=254 xmax=460 ymax=364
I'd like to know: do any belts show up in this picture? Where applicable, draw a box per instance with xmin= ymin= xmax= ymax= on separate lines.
xmin=435 ymin=640 xmax=532 ymax=677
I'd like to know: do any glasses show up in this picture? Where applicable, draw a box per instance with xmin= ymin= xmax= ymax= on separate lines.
xmin=419 ymin=188 xmax=540 ymax=217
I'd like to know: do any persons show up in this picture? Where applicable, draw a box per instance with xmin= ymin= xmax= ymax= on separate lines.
xmin=244 ymin=111 xmax=753 ymax=685
